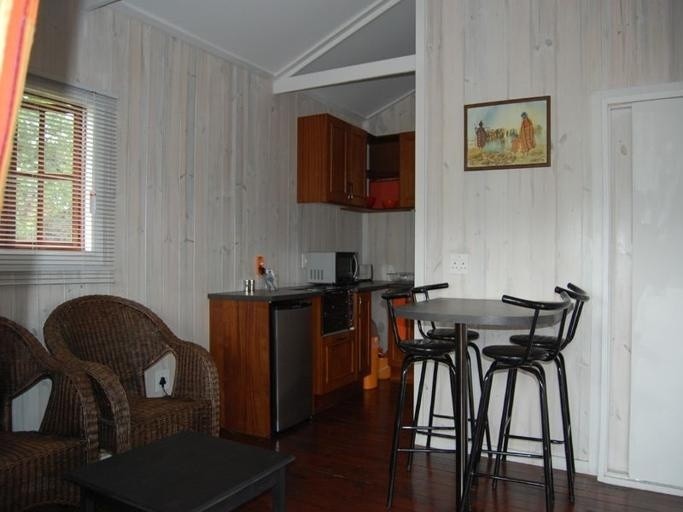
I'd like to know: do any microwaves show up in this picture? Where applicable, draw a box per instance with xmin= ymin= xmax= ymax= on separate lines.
xmin=302 ymin=251 xmax=359 ymax=284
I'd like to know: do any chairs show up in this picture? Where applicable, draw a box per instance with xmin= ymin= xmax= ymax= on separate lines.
xmin=491 ymin=282 xmax=590 ymax=504
xmin=43 ymin=295 xmax=221 ymax=455
xmin=461 ymin=290 xmax=571 ymax=512
xmin=393 ymin=297 xmax=574 ymax=512
xmin=411 ymin=284 xmax=493 ymax=485
xmin=0 ymin=315 xmax=100 ymax=512
xmin=381 ymin=282 xmax=475 ymax=511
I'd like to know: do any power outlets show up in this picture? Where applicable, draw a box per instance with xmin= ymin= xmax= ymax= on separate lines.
xmin=155 ymin=368 xmax=171 ymax=392
xmin=449 ymin=253 xmax=469 ymax=273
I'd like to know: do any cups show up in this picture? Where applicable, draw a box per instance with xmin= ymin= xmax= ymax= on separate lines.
xmin=386 ymin=271 xmax=412 ymax=283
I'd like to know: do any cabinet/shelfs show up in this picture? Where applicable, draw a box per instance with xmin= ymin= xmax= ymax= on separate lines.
xmin=207 ymin=299 xmax=315 ymax=442
xmin=314 ymin=293 xmax=365 ymax=414
xmin=296 ymin=112 xmax=367 ymax=207
xmin=366 ymin=289 xmax=413 ymax=389
xmin=367 ymin=131 xmax=415 ymax=209
